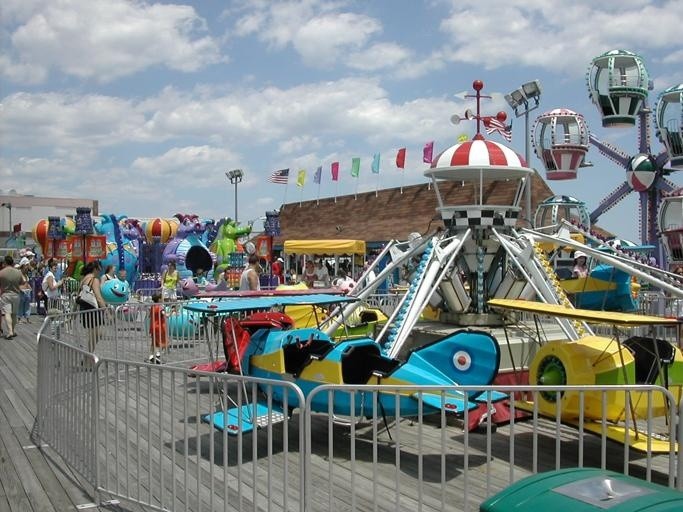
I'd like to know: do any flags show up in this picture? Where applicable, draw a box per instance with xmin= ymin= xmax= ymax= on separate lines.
xmin=311 ymin=166 xmax=321 ymax=186
xmin=270 ymin=169 xmax=288 ymax=185
xmin=349 ymin=157 xmax=359 ymax=177
xmin=395 ymin=148 xmax=404 ymax=168
xmin=369 ymin=152 xmax=380 ymax=173
xmin=331 ymin=162 xmax=338 ymax=180
xmin=421 ymin=139 xmax=433 ymax=164
xmin=295 ymin=169 xmax=305 ymax=186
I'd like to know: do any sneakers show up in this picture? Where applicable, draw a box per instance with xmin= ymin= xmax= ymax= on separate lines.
xmin=5 ymin=331 xmax=18 ymax=340
xmin=144 ymin=353 xmax=165 ymax=365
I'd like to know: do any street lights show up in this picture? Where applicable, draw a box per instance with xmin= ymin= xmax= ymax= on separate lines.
xmin=225 ymin=169 xmax=244 ymax=222
xmin=504 ymin=80 xmax=541 ymax=227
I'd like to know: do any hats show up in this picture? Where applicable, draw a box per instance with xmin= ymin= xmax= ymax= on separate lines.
xmin=25 ymin=251 xmax=36 ymax=256
xmin=573 ymin=250 xmax=588 ymax=259
xmin=19 ymin=257 xmax=31 ymax=265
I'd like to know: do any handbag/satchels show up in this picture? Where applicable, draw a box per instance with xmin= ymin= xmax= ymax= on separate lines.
xmin=75 ymin=276 xmax=99 ymax=310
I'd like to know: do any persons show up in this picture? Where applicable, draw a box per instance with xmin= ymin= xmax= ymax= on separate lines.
xmin=570 ymin=248 xmax=591 ymax=278
xmin=270 ymin=255 xmax=364 ymax=292
xmin=70 ymin=260 xmax=105 ymax=371
xmin=146 ymin=291 xmax=178 ymax=364
xmin=98 ymin=263 xmax=119 ymax=285
xmin=116 ymin=266 xmax=130 ymax=305
xmin=160 ymin=261 xmax=181 ymax=312
xmin=0 ymin=248 xmax=67 ymax=340
xmin=242 ymin=253 xmax=262 ymax=296
